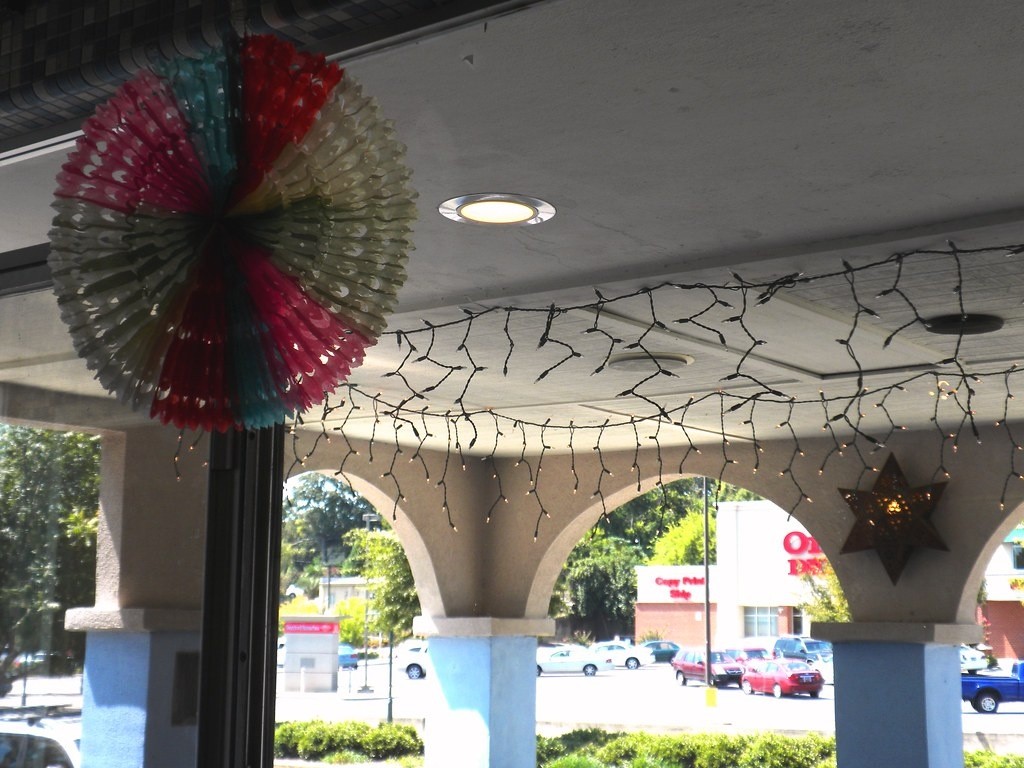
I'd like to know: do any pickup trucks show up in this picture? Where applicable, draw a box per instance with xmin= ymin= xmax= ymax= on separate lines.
xmin=961 ymin=660 xmax=1024 ymax=714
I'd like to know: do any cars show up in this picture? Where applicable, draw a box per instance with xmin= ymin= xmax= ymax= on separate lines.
xmin=739 ymin=657 xmax=824 ymax=698
xmin=669 ymin=646 xmax=745 ymax=689
xmin=637 ymin=639 xmax=681 ymax=664
xmin=722 ymin=643 xmax=774 ymax=673
xmin=958 ymin=642 xmax=988 ymax=676
xmin=396 ymin=638 xmax=429 ymax=681
xmin=337 ymin=645 xmax=358 ymax=670
xmin=0 ymin=645 xmax=53 ymax=671
xmin=531 ymin=645 xmax=617 ymax=677
xmin=587 ymin=641 xmax=656 ymax=670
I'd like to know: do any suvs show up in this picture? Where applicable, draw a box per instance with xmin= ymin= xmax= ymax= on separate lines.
xmin=771 ymin=634 xmax=834 ymax=675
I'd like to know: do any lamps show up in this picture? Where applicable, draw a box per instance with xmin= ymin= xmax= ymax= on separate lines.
xmin=836 ymin=453 xmax=950 ymax=587
